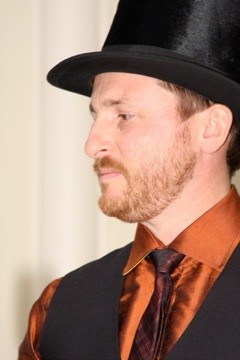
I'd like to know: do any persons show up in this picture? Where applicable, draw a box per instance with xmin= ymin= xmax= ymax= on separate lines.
xmin=19 ymin=0 xmax=240 ymax=360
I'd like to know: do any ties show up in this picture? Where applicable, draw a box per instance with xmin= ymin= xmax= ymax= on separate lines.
xmin=127 ymin=249 xmax=186 ymax=360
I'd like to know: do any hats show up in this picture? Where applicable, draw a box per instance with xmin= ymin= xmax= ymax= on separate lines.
xmin=46 ymin=0 xmax=240 ymax=115
